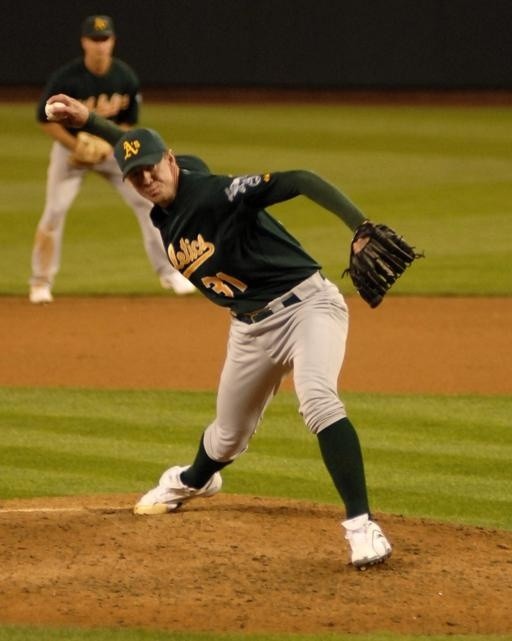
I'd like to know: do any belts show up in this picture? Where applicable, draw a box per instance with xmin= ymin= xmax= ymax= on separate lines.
xmin=236 ymin=294 xmax=301 ymax=324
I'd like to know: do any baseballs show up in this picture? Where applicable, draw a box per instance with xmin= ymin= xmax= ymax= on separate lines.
xmin=45 ymin=102 xmax=68 ymax=121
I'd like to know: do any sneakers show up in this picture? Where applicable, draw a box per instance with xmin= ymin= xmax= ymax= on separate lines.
xmin=30 ymin=286 xmax=52 ymax=303
xmin=134 ymin=466 xmax=222 ymax=514
xmin=161 ymin=272 xmax=196 ymax=294
xmin=341 ymin=513 xmax=392 ymax=566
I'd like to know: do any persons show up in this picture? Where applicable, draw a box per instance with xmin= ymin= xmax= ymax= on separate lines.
xmin=26 ymin=13 xmax=198 ymax=303
xmin=41 ymin=92 xmax=418 ymax=568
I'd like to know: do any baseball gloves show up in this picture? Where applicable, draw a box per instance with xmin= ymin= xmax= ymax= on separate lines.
xmin=73 ymin=132 xmax=113 ymax=164
xmin=350 ymin=223 xmax=414 ymax=309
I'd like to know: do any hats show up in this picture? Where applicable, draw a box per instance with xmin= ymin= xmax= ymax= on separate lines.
xmin=81 ymin=14 xmax=114 ymax=37
xmin=113 ymin=128 xmax=167 ymax=182
